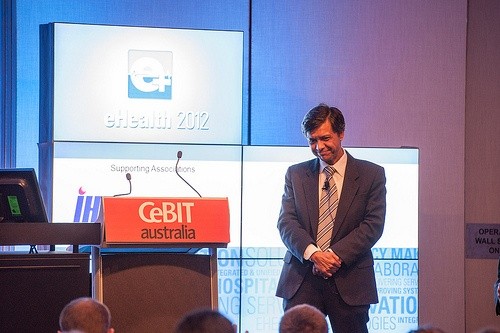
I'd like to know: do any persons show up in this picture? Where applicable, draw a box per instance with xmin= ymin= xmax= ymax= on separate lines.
xmin=274 ymin=103 xmax=387 ymax=333
xmin=57 ymin=296 xmax=115 ymax=333
xmin=279 ymin=304 xmax=329 ymax=333
xmin=174 ymin=310 xmax=237 ymax=333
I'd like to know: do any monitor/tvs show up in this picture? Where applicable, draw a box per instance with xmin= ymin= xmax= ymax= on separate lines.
xmin=0 ymin=168 xmax=48 ymax=224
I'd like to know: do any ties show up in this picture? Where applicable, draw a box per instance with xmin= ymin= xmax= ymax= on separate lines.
xmin=317 ymin=165 xmax=339 ymax=252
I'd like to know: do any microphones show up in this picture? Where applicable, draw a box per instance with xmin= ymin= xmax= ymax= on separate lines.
xmin=325 ymin=181 xmax=330 ymax=188
xmin=113 ymin=173 xmax=132 ymax=197
xmin=175 ymin=151 xmax=202 ymax=198
